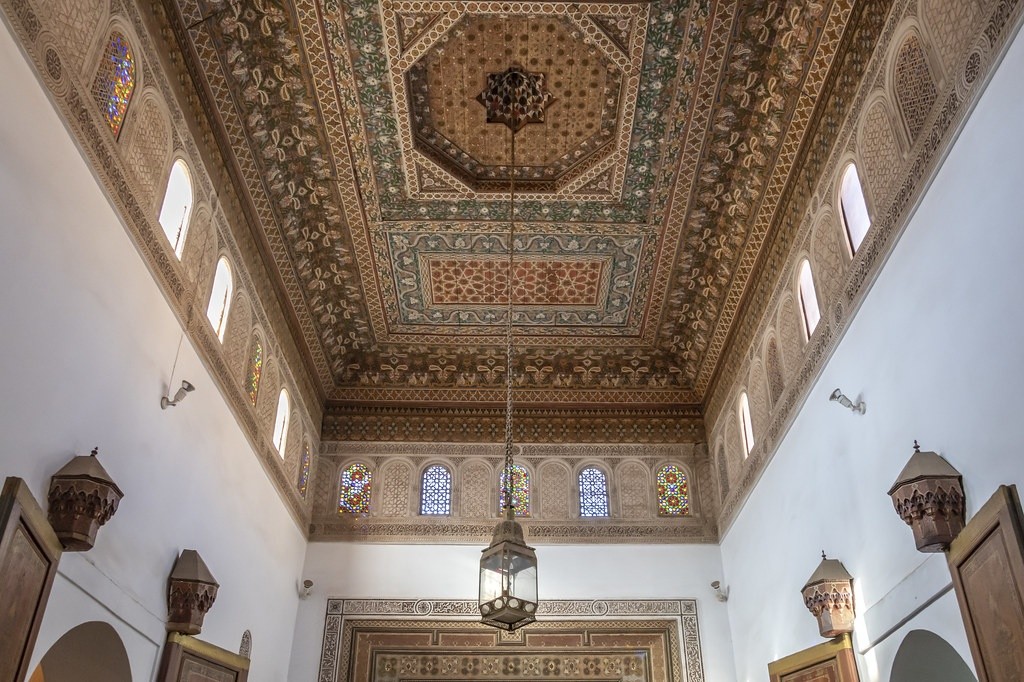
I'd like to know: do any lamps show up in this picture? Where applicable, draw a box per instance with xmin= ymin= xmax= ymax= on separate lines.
xmin=711 ymin=581 xmax=727 ymax=602
xmin=801 ymin=550 xmax=857 ymax=639
xmin=300 ymin=579 xmax=314 ymax=600
xmin=163 ymin=549 xmax=220 ymax=635
xmin=887 ymin=439 xmax=966 ymax=554
xmin=160 ymin=380 xmax=195 ymax=409
xmin=47 ymin=445 xmax=125 ymax=553
xmin=828 ymin=388 xmax=866 ymax=416
xmin=478 ymin=73 xmax=539 ymax=634
